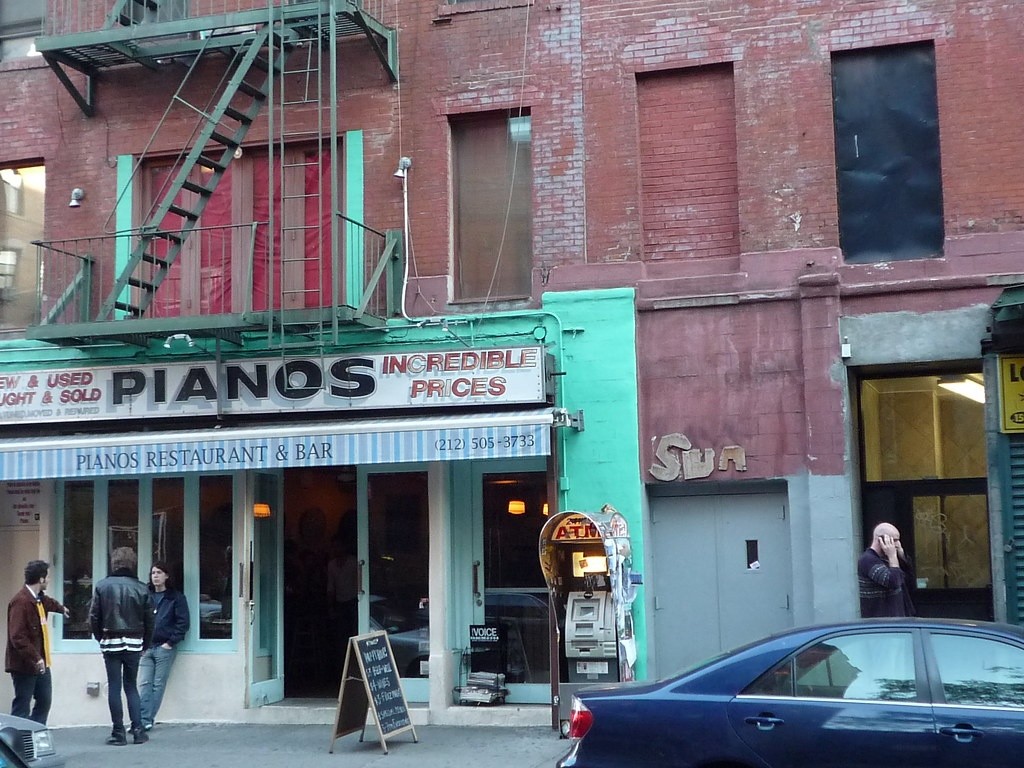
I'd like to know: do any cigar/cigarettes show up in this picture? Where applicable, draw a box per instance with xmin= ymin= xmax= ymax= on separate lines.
xmin=39 ymin=669 xmax=43 ymax=672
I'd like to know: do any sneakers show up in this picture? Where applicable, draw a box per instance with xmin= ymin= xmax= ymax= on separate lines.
xmin=133 ymin=728 xmax=149 ymax=744
xmin=103 ymin=729 xmax=127 ymax=747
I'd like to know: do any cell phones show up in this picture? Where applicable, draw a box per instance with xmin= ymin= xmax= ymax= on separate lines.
xmin=878 ymin=535 xmax=885 ymax=546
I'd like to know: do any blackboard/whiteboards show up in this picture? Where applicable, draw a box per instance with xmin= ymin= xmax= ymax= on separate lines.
xmin=353 ymin=631 xmax=414 ymax=737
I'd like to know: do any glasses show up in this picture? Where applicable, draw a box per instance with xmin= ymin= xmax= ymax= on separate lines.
xmin=876 ymin=535 xmax=901 ymax=546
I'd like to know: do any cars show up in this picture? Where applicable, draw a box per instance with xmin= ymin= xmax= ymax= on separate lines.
xmin=200 ymin=593 xmax=223 ymax=623
xmin=484 ymin=594 xmax=564 ymax=679
xmin=369 ymin=619 xmax=429 ymax=678
xmin=557 ymin=617 xmax=1024 ymax=768
xmin=0 ymin=713 xmax=64 ymax=768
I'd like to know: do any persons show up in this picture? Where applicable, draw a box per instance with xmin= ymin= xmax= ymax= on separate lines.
xmin=90 ymin=547 xmax=154 ymax=745
xmin=857 ymin=522 xmax=916 ymax=619
xmin=125 ymin=562 xmax=190 ymax=734
xmin=5 ymin=560 xmax=69 ymax=726
